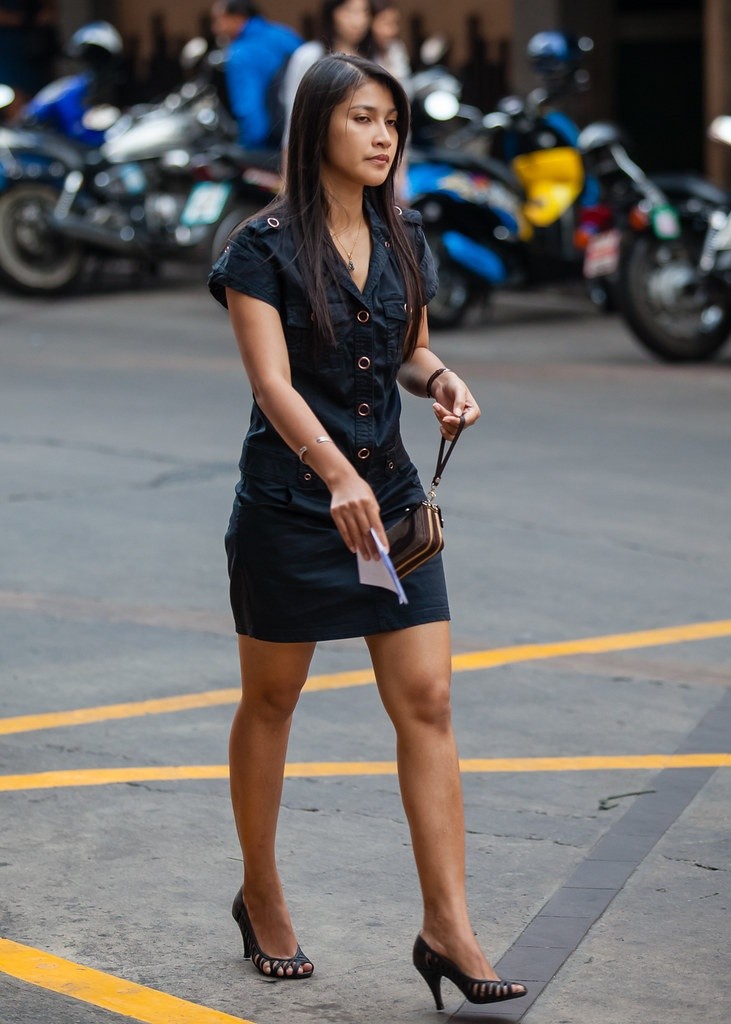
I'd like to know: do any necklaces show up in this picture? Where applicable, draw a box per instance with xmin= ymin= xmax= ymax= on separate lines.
xmin=333 ymin=221 xmax=362 ymax=270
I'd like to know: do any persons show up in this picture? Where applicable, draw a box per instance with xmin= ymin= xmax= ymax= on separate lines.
xmin=211 ymin=0 xmax=303 ymax=151
xmin=207 ymin=54 xmax=530 ymax=1010
xmin=279 ymin=0 xmax=411 ymax=206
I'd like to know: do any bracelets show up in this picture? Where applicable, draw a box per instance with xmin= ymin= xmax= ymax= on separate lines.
xmin=426 ymin=368 xmax=451 ymax=397
xmin=297 ymin=436 xmax=337 ymax=464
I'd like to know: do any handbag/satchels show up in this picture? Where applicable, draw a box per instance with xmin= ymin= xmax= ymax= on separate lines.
xmin=385 ymin=417 xmax=464 ymax=580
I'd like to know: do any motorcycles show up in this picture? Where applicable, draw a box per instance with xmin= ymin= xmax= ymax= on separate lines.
xmin=1 ymin=19 xmax=731 ymax=364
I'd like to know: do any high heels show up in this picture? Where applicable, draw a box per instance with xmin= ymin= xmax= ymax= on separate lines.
xmin=413 ymin=934 xmax=527 ymax=1010
xmin=232 ymin=885 xmax=314 ymax=978
